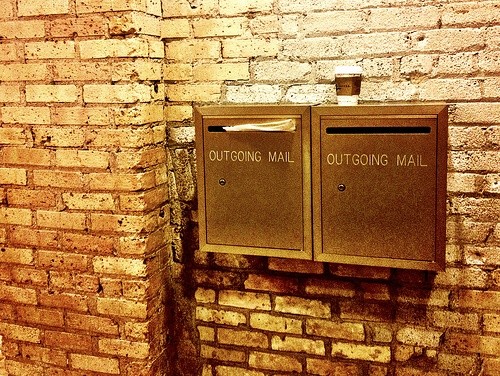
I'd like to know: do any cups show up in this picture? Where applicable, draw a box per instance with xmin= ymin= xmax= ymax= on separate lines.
xmin=332 ymin=65 xmax=364 ymax=105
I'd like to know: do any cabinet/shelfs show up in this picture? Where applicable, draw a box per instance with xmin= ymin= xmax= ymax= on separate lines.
xmin=193 ymin=103 xmax=453 ymax=276
